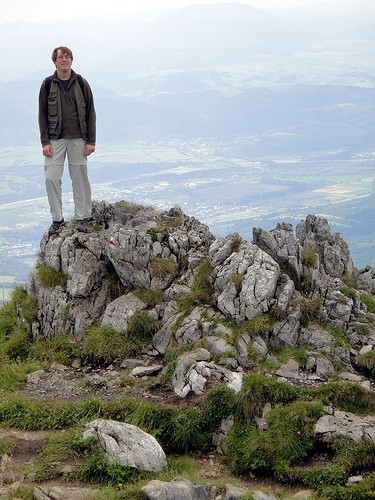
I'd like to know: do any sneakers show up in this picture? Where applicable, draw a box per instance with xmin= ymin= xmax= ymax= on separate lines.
xmin=77 ymin=217 xmax=86 ymax=232
xmin=49 ymin=217 xmax=65 ymax=234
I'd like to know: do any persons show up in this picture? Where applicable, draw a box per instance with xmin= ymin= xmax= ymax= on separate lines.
xmin=38 ymin=46 xmax=96 ymax=233
